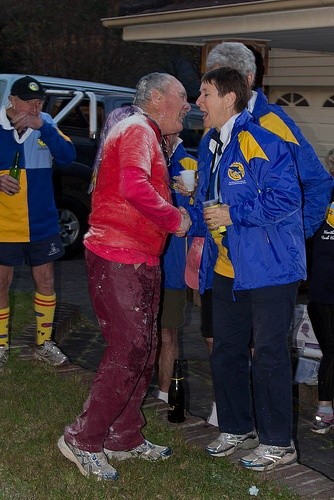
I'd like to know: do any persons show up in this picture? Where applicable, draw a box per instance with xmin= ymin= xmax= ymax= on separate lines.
xmin=57 ymin=73 xmax=192 ymax=481
xmin=155 ymin=132 xmax=199 ymax=404
xmin=174 ymin=41 xmax=334 ymax=427
xmin=306 ymin=201 xmax=334 ymax=434
xmin=172 ymin=65 xmax=307 ymax=472
xmin=0 ymin=76 xmax=77 ymax=368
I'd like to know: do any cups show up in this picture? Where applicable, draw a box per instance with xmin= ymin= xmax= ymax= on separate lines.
xmin=179 ymin=169 xmax=196 ymax=192
xmin=203 ymin=199 xmax=219 ymax=231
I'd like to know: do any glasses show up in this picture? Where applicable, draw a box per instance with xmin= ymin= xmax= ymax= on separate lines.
xmin=162 ymin=139 xmax=169 ymax=164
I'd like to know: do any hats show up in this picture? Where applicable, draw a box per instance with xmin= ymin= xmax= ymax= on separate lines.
xmin=9 ymin=76 xmax=48 ymax=102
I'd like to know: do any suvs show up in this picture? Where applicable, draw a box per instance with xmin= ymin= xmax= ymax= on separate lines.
xmin=1 ymin=74 xmax=207 ymax=260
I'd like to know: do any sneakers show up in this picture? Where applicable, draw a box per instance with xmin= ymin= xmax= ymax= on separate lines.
xmin=205 ymin=431 xmax=259 ymax=457
xmin=237 ymin=439 xmax=299 ymax=473
xmin=57 ymin=434 xmax=118 ymax=481
xmin=103 ymin=439 xmax=172 ymax=463
xmin=311 ymin=412 xmax=334 ymax=434
xmin=0 ymin=348 xmax=10 ymax=366
xmin=34 ymin=340 xmax=68 ymax=366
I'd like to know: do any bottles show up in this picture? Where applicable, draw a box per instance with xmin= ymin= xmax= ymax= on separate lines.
xmin=168 ymin=359 xmax=185 ymax=424
xmin=8 ymin=152 xmax=21 ymax=193
